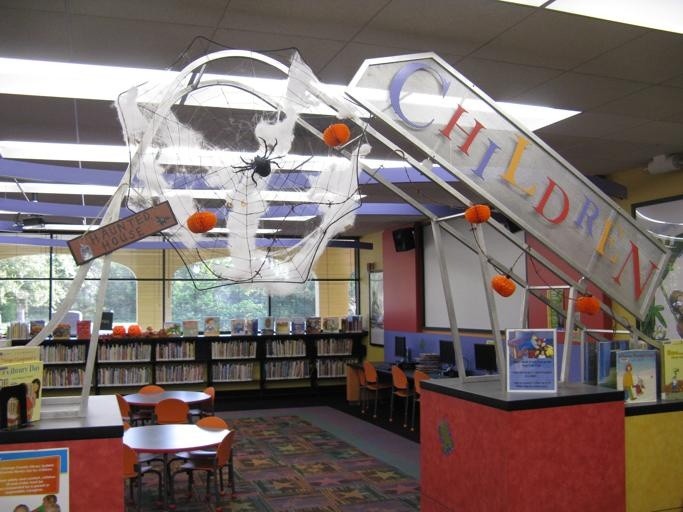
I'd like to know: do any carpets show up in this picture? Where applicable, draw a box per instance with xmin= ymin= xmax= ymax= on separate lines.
xmin=122 ymin=416 xmax=422 ymax=511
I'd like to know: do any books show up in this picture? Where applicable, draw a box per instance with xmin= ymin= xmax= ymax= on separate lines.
xmin=415 ymin=352 xmax=441 ymax=373
xmin=211 ymin=341 xmax=257 ymax=379
xmin=95 ymin=342 xmax=152 ymax=385
xmin=164 ymin=315 xmax=363 ymax=337
xmin=153 ymin=342 xmax=208 ymax=382
xmin=315 ymin=339 xmax=358 ymax=378
xmin=264 ymin=339 xmax=306 ymax=378
xmin=1 ymin=320 xmax=87 ymax=388
xmin=504 ymin=329 xmax=682 ymax=404
xmin=1 ymin=346 xmax=44 ymax=426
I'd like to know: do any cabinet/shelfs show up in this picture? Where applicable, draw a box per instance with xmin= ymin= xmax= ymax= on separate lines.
xmin=11 ymin=329 xmax=367 ymax=399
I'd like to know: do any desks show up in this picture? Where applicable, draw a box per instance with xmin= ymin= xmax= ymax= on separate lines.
xmin=0 ymin=392 xmax=126 ymax=512
xmin=420 ymin=375 xmax=624 ymax=512
xmin=624 ymin=396 xmax=682 ymax=512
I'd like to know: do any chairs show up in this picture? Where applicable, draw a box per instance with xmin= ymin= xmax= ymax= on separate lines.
xmin=341 ymin=354 xmax=448 ymax=434
xmin=115 ymin=387 xmax=234 ymax=512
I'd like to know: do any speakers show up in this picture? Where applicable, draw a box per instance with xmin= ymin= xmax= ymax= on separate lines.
xmin=392 ymin=227 xmax=415 ymax=252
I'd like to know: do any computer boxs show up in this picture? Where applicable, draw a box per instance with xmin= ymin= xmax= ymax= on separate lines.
xmin=390 ymin=360 xmax=420 ymax=370
xmin=438 ymin=369 xmax=471 ymax=377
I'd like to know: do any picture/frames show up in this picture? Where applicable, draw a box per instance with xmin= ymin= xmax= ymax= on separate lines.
xmin=503 ymin=327 xmax=557 ymax=395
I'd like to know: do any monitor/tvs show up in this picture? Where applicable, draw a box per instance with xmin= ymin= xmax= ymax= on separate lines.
xmin=439 ymin=340 xmax=456 ymax=370
xmin=475 ymin=344 xmax=498 ymax=371
xmin=395 ymin=336 xmax=409 ymax=361
